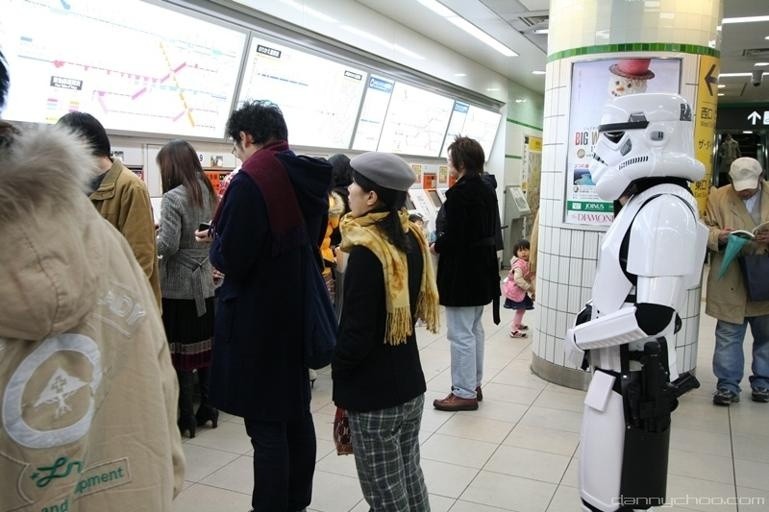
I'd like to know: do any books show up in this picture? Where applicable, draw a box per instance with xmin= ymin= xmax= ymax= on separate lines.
xmin=728 ymin=220 xmax=769 ymax=240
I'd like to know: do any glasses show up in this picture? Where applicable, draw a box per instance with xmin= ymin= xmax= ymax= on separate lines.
xmin=231 ymin=139 xmax=241 ymax=158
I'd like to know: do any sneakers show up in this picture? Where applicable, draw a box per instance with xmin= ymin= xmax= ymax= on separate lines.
xmin=509 ymin=331 xmax=528 ymax=338
xmin=751 ymin=386 xmax=769 ymax=402
xmin=713 ymin=389 xmax=740 ymax=406
xmin=434 ymin=393 xmax=478 ymax=411
xmin=518 ymin=325 xmax=528 ymax=330
xmin=452 ymin=386 xmax=483 ymax=401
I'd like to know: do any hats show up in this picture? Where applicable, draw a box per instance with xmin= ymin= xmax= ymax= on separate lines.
xmin=728 ymin=157 xmax=763 ymax=192
xmin=350 ymin=151 xmax=418 ymax=192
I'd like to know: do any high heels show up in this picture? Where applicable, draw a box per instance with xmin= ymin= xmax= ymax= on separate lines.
xmin=177 ymin=416 xmax=198 ymax=439
xmin=195 ymin=404 xmax=219 ymax=428
xmin=309 ymin=368 xmax=318 ymax=388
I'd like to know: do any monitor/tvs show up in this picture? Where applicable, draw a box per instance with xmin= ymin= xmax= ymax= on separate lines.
xmin=427 ymin=189 xmax=444 ymax=210
xmin=405 ymin=192 xmax=416 ymax=210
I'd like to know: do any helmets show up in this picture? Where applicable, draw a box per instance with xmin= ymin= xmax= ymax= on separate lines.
xmin=587 ymin=92 xmax=706 ymax=202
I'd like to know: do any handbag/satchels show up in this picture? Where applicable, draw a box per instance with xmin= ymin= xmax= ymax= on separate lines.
xmin=736 ymin=254 xmax=769 ymax=302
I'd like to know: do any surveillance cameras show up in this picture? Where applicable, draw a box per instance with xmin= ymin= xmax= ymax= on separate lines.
xmin=751 ymin=69 xmax=764 ymax=87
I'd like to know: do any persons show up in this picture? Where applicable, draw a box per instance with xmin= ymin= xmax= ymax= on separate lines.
xmin=0 ymin=50 xmax=189 ymax=512
xmin=704 ymin=156 xmax=768 ymax=410
xmin=500 ymin=237 xmax=535 ymax=341
xmin=425 ymin=133 xmax=505 ymax=414
xmin=61 ymin=94 xmax=435 ymax=511
xmin=559 ymin=89 xmax=709 ymax=512
xmin=717 ymin=132 xmax=743 ymax=188
